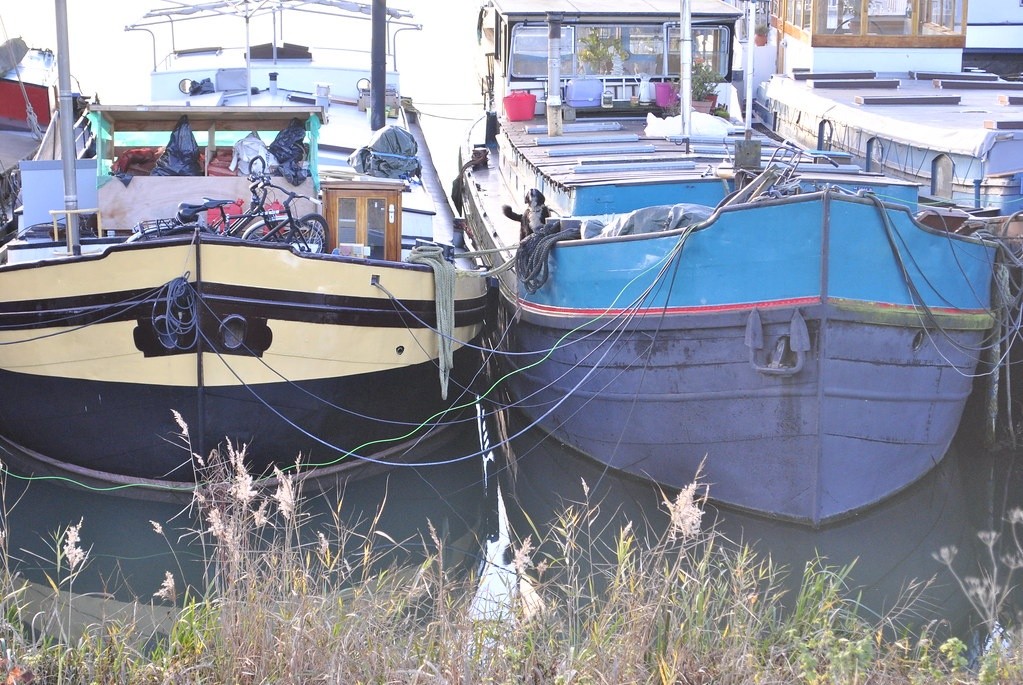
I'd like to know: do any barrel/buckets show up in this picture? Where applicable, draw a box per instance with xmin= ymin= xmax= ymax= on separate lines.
xmin=207 ymin=198 xmax=244 ymax=234
xmin=654 ymin=80 xmax=679 ymax=108
xmin=260 ymin=201 xmax=285 ymax=242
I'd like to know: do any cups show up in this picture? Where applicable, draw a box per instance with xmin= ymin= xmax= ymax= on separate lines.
xmin=631 ymin=96 xmax=639 ymax=108
xmin=599 ymin=91 xmax=613 ymax=109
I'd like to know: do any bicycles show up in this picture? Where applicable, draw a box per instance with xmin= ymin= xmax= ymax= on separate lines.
xmin=123 ymin=155 xmax=328 ymax=257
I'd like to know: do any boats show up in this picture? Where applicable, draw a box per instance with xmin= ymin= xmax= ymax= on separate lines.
xmin=0 ymin=0 xmax=489 ymax=490
xmin=449 ymin=0 xmax=999 ymax=527
xmin=735 ymin=0 xmax=1023 ymax=255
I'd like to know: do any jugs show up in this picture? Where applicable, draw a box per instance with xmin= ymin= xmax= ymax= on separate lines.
xmin=633 ymin=73 xmax=653 ymax=106
